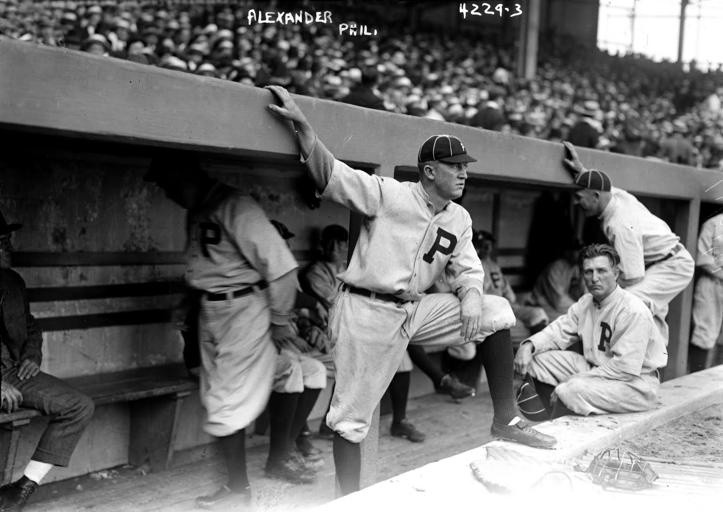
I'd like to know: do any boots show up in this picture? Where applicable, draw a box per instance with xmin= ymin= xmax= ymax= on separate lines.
xmin=0 ymin=475 xmax=38 ymax=512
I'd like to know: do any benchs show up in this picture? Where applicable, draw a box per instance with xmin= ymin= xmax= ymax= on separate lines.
xmin=1 ymin=247 xmax=542 ymax=511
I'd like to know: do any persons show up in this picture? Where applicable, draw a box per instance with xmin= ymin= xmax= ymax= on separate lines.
xmin=183 ymin=218 xmax=329 ymax=484
xmin=0 ymin=214 xmax=96 ymax=512
xmin=260 ymin=83 xmax=561 ymax=498
xmin=506 ymin=244 xmax=668 ymax=418
xmin=692 ymin=212 xmax=723 ymax=373
xmin=562 ymin=139 xmax=697 ymax=370
xmin=532 ymin=240 xmax=582 ymax=323
xmin=302 ymin=224 xmax=477 ymax=443
xmin=156 ymin=159 xmax=315 ymax=510
xmin=2 ymin=2 xmax=723 ymax=168
xmin=438 ymin=340 xmax=480 ymax=384
xmin=479 ymin=230 xmax=548 ymax=333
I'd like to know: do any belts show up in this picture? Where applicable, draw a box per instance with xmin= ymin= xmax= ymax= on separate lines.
xmin=338 ymin=283 xmax=410 ymax=306
xmin=644 ymin=244 xmax=682 ymax=271
xmin=206 ymin=279 xmax=269 ymax=301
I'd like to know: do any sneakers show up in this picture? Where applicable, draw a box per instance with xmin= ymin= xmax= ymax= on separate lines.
xmin=433 ymin=374 xmax=476 ymax=400
xmin=196 ymin=484 xmax=251 ymax=509
xmin=390 ymin=419 xmax=426 ymax=442
xmin=265 ymin=447 xmax=324 ymax=483
xmin=491 ymin=416 xmax=558 ymax=449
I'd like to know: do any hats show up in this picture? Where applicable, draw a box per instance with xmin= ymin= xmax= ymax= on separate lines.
xmin=515 ymin=372 xmax=551 ymax=422
xmin=269 ymin=219 xmax=295 ymax=239
xmin=418 ymin=135 xmax=478 ymax=163
xmin=477 ymin=231 xmax=497 ymax=244
xmin=566 ymin=169 xmax=611 ymax=192
